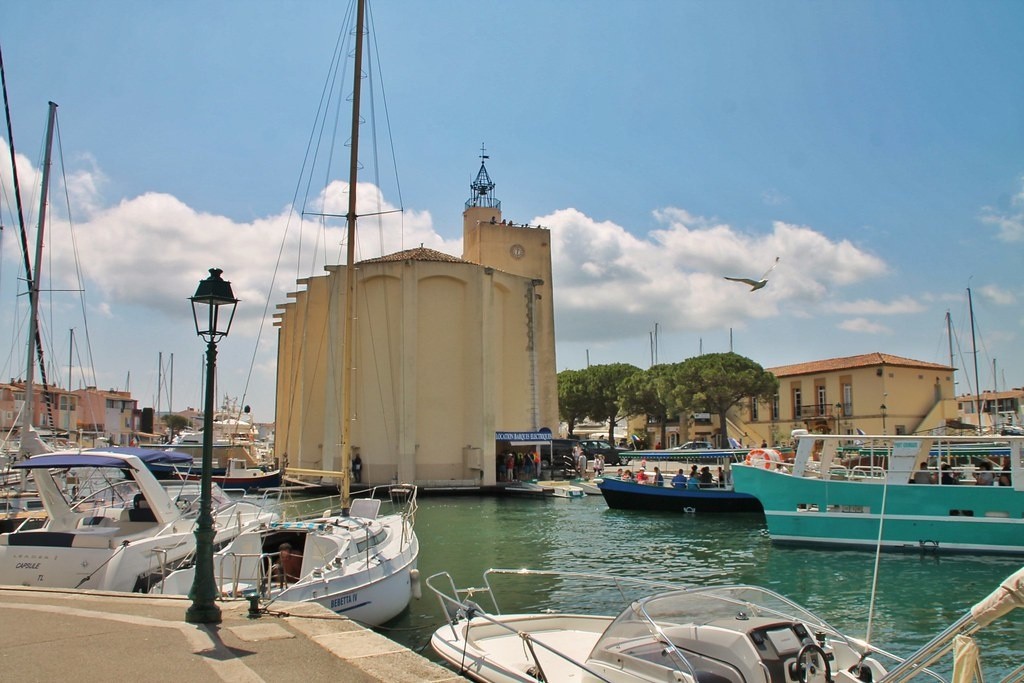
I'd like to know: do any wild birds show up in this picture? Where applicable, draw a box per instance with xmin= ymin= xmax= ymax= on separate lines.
xmin=724 ymin=257 xmax=782 ymax=292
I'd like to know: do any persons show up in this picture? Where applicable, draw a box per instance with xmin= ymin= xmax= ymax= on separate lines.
xmin=274 ymin=543 xmax=302 ymax=584
xmin=913 ymin=462 xmax=1012 ymax=485
xmin=761 ymin=439 xmax=780 ymax=448
xmin=572 ymin=442 xmax=724 ymax=491
xmin=497 ymin=450 xmax=536 ymax=482
xmin=352 ymin=452 xmax=362 ymax=482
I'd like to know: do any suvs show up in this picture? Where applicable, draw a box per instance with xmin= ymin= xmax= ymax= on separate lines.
xmin=580 ymin=440 xmax=633 ymax=466
xmin=667 ymin=441 xmax=713 ymax=450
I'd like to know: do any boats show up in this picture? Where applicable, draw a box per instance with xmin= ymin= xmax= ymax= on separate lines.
xmin=0 ymin=446 xmax=280 ymax=597
xmin=569 ymin=479 xmax=602 ymax=495
xmin=527 ymin=479 xmax=587 ymax=499
xmin=730 ymin=428 xmax=1024 ymax=556
xmin=593 ymin=449 xmax=790 ymax=510
xmin=1 ymin=46 xmax=281 ymax=529
xmin=426 ymin=568 xmax=1024 ymax=683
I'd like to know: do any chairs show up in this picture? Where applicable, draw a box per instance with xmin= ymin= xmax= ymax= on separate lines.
xmin=129 ymin=494 xmax=157 ymax=522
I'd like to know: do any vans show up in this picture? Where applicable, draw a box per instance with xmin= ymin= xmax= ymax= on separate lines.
xmin=526 ymin=439 xmax=593 ymax=470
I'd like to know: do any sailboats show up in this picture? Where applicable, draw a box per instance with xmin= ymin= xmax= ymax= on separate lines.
xmin=97 ymin=0 xmax=417 ymax=630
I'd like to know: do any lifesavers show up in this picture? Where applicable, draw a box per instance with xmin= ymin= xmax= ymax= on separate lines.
xmin=746 ymin=449 xmax=770 ymax=469
xmin=518 ymin=453 xmax=523 ymax=459
xmin=773 ymin=450 xmax=782 ymax=460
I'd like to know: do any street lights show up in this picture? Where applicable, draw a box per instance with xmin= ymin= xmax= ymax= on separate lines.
xmin=835 ymin=402 xmax=842 ymax=446
xmin=880 ymin=404 xmax=887 ymax=434
xmin=185 ymin=267 xmax=240 ymax=625
